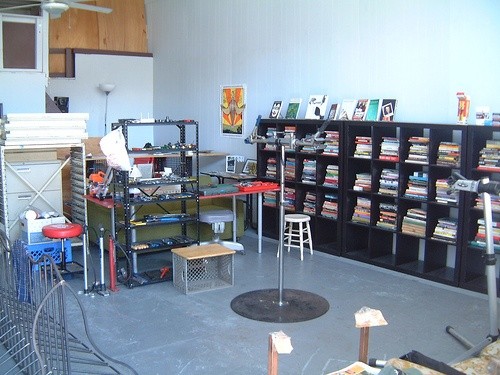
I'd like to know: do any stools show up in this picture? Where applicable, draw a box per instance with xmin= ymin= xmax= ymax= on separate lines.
xmin=42 ymin=223 xmax=88 ymax=277
xmin=276 ymin=214 xmax=313 ymax=261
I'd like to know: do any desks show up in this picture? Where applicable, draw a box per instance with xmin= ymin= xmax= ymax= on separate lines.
xmin=82 ymin=182 xmax=281 ymax=274
xmin=200 ymin=171 xmax=257 ymax=231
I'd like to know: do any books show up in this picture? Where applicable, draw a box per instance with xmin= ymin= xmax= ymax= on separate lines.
xmin=320 ymin=193 xmax=338 ymax=220
xmin=402 ymin=207 xmax=426 ymax=239
xmin=321 ymin=164 xmax=338 ymax=188
xmin=404 ymin=170 xmax=428 ymax=201
xmin=471 ymin=139 xmax=500 ymax=251
xmin=301 ymin=158 xmax=318 ymax=185
xmin=379 ymin=167 xmax=398 ymax=196
xmin=266 ymin=157 xmax=277 ymax=178
xmin=378 ymin=136 xmax=400 ymax=162
xmin=303 ymin=190 xmax=316 ymax=215
xmin=376 ymin=203 xmax=397 ymax=229
xmin=351 ymin=196 xmax=372 ymax=224
xmin=285 ymin=157 xmax=296 ymax=181
xmin=263 ymin=125 xmax=296 ymax=151
xmin=0 ymin=113 xmax=90 ymax=147
xmin=269 ymin=94 xmax=397 ymax=122
xmin=299 ymin=130 xmax=339 ymax=154
xmin=354 ymin=136 xmax=373 ymax=158
xmin=263 ymin=185 xmax=295 ymax=211
xmin=353 ymin=171 xmax=371 ymax=192
xmin=432 ymin=142 xmax=461 ymax=242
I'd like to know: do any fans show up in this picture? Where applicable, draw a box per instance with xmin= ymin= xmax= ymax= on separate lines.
xmin=0 ymin=0 xmax=113 ymax=19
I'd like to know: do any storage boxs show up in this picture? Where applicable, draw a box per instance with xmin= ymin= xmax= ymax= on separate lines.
xmin=15 ymin=216 xmax=72 ymax=271
xmin=171 ymin=243 xmax=236 ymax=295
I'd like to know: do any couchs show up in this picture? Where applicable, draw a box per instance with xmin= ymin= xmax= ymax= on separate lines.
xmin=86 ymin=175 xmax=244 ymax=258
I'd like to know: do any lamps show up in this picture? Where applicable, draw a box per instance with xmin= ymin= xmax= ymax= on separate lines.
xmin=99 ymin=82 xmax=116 ymax=134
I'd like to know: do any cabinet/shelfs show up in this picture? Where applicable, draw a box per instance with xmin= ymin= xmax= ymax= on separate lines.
xmin=111 ymin=119 xmax=201 ymax=288
xmin=256 ymin=119 xmax=500 ymax=298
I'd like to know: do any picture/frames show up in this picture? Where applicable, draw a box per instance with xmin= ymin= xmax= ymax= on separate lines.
xmin=220 ymin=85 xmax=246 ymax=138
